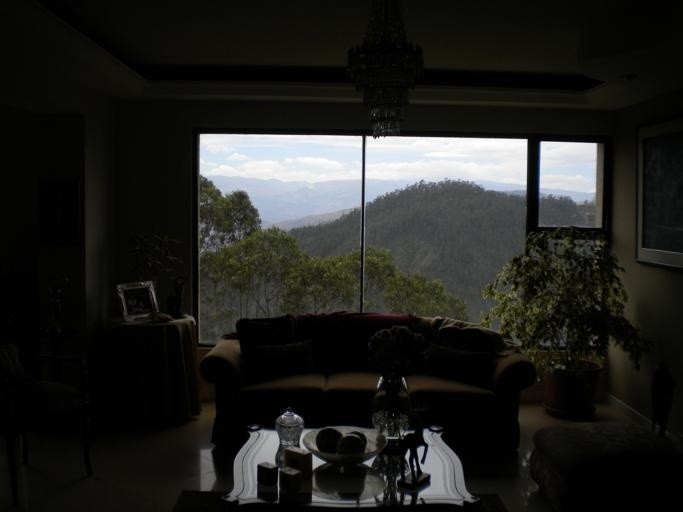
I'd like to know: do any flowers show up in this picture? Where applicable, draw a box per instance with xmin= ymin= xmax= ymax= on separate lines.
xmin=361 ymin=326 xmax=426 ymax=465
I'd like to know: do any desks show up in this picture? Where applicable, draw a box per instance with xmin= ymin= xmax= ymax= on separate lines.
xmin=106 ymin=316 xmax=198 ymax=424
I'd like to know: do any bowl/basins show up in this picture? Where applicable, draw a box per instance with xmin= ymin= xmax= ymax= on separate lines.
xmin=311 ymin=463 xmax=388 ymax=501
xmin=304 ymin=424 xmax=388 ymax=465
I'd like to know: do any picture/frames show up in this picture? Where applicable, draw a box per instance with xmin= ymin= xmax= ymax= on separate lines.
xmin=634 ymin=113 xmax=683 ymax=272
xmin=115 ymin=279 xmax=159 ymax=322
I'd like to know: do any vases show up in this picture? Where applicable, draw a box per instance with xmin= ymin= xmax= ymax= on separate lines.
xmin=371 ymin=379 xmax=413 ymax=473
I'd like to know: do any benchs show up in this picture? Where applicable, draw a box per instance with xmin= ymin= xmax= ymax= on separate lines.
xmin=530 ymin=421 xmax=683 ymax=512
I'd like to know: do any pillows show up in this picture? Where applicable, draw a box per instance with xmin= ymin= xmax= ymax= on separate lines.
xmin=428 ymin=326 xmax=492 ymax=386
xmin=237 ymin=315 xmax=314 ymax=381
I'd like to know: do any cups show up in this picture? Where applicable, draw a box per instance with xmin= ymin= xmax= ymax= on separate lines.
xmin=275 ymin=444 xmax=301 ymax=469
xmin=276 ymin=406 xmax=304 ymax=447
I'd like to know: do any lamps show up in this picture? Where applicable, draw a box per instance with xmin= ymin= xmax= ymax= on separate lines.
xmin=346 ymin=0 xmax=424 ymax=135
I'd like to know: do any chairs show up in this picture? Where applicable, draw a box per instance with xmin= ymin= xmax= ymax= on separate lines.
xmin=0 ymin=341 xmax=89 ymax=510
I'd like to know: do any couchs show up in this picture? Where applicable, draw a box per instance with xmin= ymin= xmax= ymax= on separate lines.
xmin=199 ymin=311 xmax=537 ymax=448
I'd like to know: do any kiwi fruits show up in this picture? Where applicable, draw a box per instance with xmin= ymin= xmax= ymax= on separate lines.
xmin=347 ymin=431 xmax=367 ymax=446
xmin=316 ymin=428 xmax=342 ymax=453
xmin=336 ymin=435 xmax=365 ymax=454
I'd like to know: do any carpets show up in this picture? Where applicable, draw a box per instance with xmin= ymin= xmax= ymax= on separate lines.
xmin=171 ymin=490 xmax=508 ymax=512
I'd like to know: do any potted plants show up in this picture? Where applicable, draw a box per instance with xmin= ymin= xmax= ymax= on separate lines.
xmin=477 ymin=226 xmax=655 ymax=419
